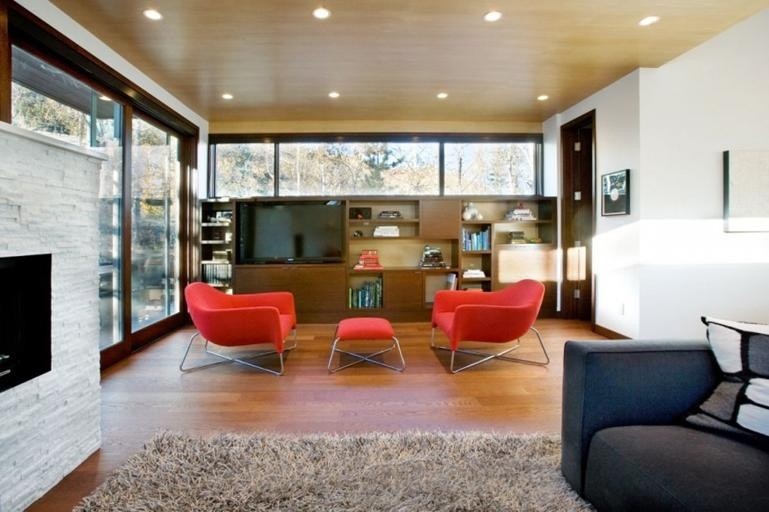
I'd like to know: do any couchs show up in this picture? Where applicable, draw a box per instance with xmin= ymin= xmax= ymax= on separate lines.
xmin=552 ymin=318 xmax=769 ymax=510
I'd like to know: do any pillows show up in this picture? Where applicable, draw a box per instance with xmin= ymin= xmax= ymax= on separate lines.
xmin=671 ymin=312 xmax=769 ymax=453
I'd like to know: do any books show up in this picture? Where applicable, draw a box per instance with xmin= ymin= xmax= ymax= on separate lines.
xmin=349 ymin=203 xmax=405 ymax=311
xmin=502 ymin=204 xmax=538 ymax=243
xmin=415 ymin=243 xmax=458 ymax=290
xmin=204 ymin=265 xmax=235 ymax=288
xmin=462 ymin=200 xmax=492 ymax=291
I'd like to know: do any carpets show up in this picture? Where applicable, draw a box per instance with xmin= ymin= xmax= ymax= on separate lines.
xmin=66 ymin=427 xmax=597 ymax=511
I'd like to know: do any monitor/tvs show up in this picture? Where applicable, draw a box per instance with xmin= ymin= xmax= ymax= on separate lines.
xmin=235 ymin=200 xmax=346 ymax=265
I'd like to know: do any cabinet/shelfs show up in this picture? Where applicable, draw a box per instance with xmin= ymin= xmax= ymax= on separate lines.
xmin=346 ymin=197 xmax=462 ymax=321
xmin=459 ymin=193 xmax=558 ymax=320
xmin=196 ymin=196 xmax=234 ymax=265
xmin=231 ymin=261 xmax=347 ymax=317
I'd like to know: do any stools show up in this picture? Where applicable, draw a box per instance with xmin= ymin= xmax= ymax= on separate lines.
xmin=324 ymin=315 xmax=408 ymax=376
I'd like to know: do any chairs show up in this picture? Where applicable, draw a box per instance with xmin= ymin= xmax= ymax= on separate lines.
xmin=428 ymin=276 xmax=552 ymax=376
xmin=177 ymin=280 xmax=300 ymax=378
xmin=185 ymin=258 xmax=235 ymax=314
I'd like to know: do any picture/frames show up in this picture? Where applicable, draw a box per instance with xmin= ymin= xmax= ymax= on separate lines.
xmin=599 ymin=169 xmax=631 ymax=217
